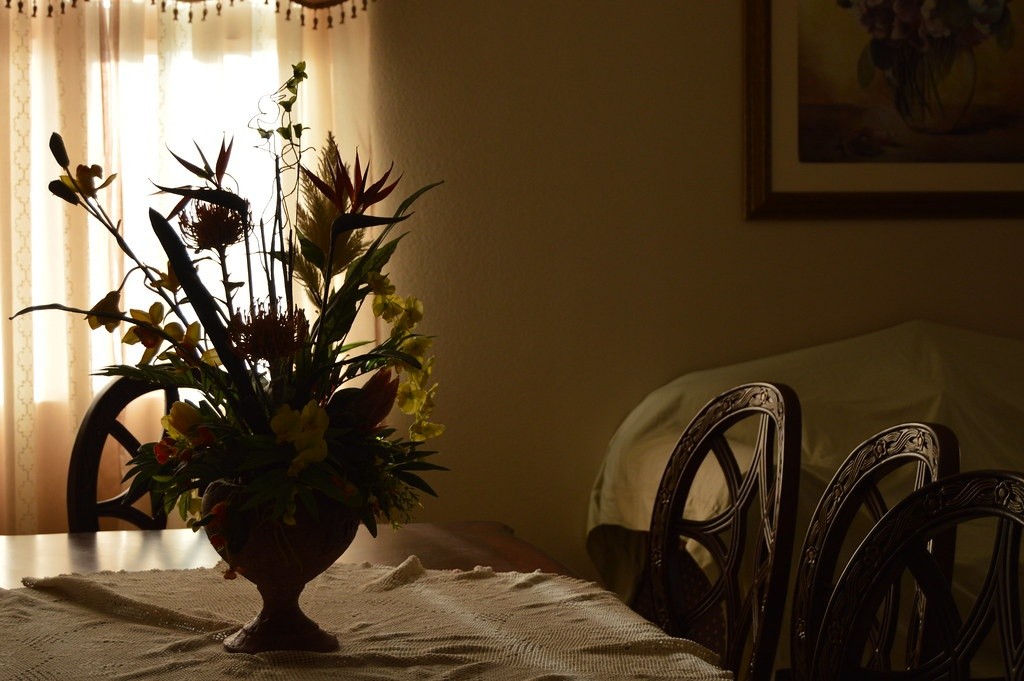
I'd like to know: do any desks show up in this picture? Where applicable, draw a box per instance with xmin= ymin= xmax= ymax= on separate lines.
xmin=1 ymin=520 xmax=738 ymax=681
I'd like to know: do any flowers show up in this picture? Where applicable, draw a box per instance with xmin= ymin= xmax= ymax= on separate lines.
xmin=9 ymin=61 xmax=451 ymax=581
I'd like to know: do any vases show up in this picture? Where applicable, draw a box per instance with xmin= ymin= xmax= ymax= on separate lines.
xmin=200 ymin=478 xmax=367 ymax=653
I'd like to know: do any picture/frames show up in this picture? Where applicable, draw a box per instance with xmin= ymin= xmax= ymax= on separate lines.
xmin=740 ymin=0 xmax=1024 ymax=221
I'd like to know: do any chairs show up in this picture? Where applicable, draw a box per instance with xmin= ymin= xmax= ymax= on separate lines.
xmin=789 ymin=423 xmax=960 ymax=680
xmin=648 ymin=382 xmax=801 ymax=680
xmin=812 ymin=469 xmax=1024 ymax=680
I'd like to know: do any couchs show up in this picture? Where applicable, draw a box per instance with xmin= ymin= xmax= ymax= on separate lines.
xmin=584 ymin=322 xmax=1024 ymax=681
